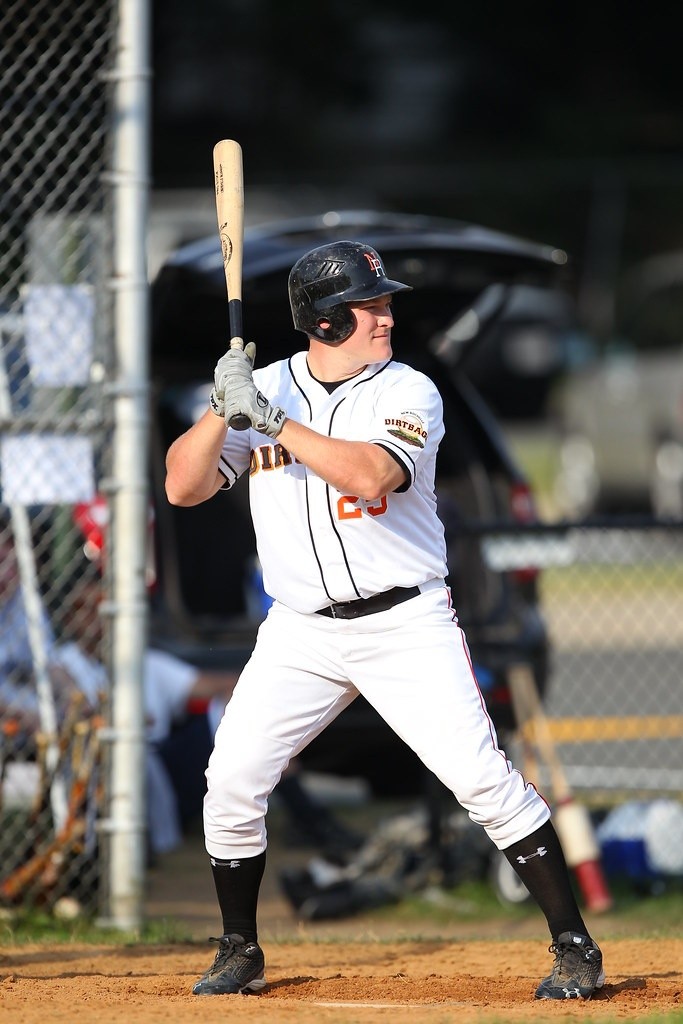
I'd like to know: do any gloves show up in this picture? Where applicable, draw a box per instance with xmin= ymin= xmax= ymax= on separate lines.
xmin=223 ymin=375 xmax=286 ymax=440
xmin=209 ymin=341 xmax=257 ymax=416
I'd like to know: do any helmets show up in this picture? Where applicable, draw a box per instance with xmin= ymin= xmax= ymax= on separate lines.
xmin=288 ymin=240 xmax=413 ymax=343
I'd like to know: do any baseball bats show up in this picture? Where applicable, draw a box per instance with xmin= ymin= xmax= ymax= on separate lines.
xmin=211 ymin=139 xmax=253 ymax=431
xmin=489 ymin=674 xmax=543 ymax=908
xmin=517 ymin=665 xmax=615 ymax=916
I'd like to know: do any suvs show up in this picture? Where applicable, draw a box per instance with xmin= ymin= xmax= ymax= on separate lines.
xmin=0 ymin=210 xmax=575 ymax=849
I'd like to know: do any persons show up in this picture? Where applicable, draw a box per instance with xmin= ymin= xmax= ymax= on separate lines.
xmin=164 ymin=237 xmax=607 ymax=1006
xmin=49 ymin=567 xmax=249 ymax=866
xmin=1 ymin=510 xmax=83 ymax=727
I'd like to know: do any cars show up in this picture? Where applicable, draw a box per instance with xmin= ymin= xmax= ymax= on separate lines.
xmin=539 ymin=247 xmax=683 ymax=512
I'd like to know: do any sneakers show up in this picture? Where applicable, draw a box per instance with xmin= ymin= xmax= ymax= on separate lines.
xmin=534 ymin=931 xmax=606 ymax=999
xmin=193 ymin=932 xmax=265 ymax=996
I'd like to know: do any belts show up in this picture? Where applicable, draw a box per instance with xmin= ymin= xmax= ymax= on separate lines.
xmin=313 ymin=586 xmax=421 ymax=620
